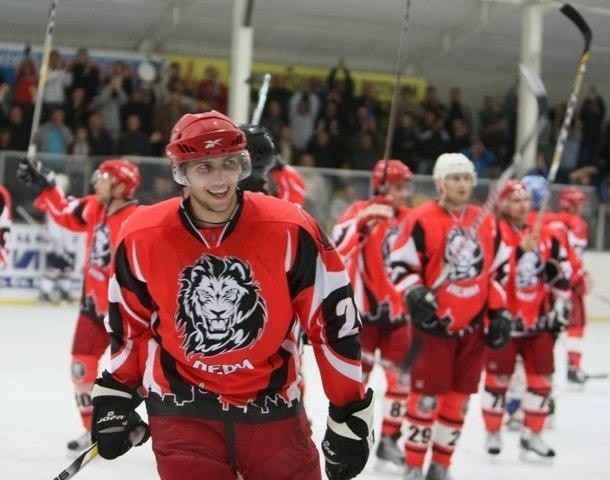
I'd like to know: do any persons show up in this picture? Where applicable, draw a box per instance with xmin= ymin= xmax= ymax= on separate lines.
xmin=1 ymin=46 xmax=600 ymax=307
xmin=18 ymin=110 xmax=600 ymax=480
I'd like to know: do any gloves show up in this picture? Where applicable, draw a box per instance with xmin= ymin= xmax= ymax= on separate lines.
xmin=377 ymin=302 xmax=407 ymax=329
xmin=547 ymin=297 xmax=572 ymax=331
xmin=91 ymin=369 xmax=136 ymax=459
xmin=321 ymin=387 xmax=375 ymax=479
xmin=484 ymin=308 xmax=513 ymax=347
xmin=403 ymin=284 xmax=438 ymax=321
xmin=16 ymin=157 xmax=56 ymax=191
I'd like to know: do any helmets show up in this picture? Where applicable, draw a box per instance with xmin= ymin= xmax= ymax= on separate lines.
xmin=494 ymin=174 xmax=586 ymax=215
xmin=373 ymin=158 xmax=413 ymax=198
xmin=164 ymin=109 xmax=253 ymax=188
xmin=432 ymin=152 xmax=478 ymax=195
xmin=89 ymin=159 xmax=142 ymax=198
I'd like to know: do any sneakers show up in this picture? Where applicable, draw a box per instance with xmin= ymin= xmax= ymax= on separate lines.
xmin=377 ymin=432 xmax=405 ymax=465
xmin=487 ymin=432 xmax=500 ymax=454
xmin=426 ymin=460 xmax=452 ymax=480
xmin=68 ymin=431 xmax=93 ymax=450
xmin=521 ymin=432 xmax=555 ymax=457
xmin=403 ymin=465 xmax=423 ymax=480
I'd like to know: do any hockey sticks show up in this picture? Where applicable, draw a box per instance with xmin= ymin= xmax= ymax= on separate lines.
xmin=531 ymin=4 xmax=594 ymax=233
xmin=423 ymin=65 xmax=546 ymax=301
